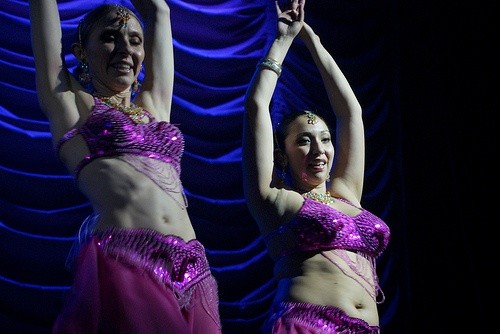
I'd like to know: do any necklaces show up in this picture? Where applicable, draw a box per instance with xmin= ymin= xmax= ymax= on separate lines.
xmin=292 ymin=188 xmax=334 ymax=204
xmin=90 ymin=96 xmax=144 ymax=120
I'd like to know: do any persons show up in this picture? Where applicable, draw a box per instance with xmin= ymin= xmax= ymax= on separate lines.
xmin=242 ymin=0 xmax=391 ymax=334
xmin=27 ymin=0 xmax=222 ymax=333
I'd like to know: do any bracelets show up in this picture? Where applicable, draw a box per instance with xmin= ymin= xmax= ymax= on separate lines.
xmin=257 ymin=57 xmax=283 ymax=77
